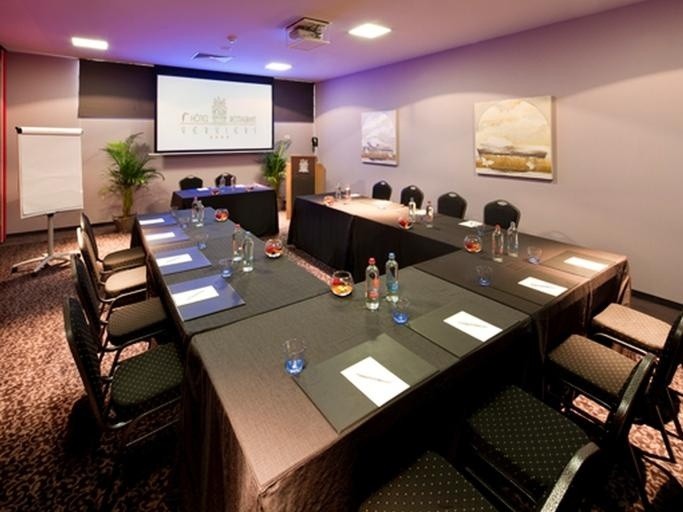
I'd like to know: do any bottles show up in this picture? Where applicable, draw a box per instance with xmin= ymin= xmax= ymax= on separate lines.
xmin=364 ymin=258 xmax=380 ymax=310
xmin=507 ymin=222 xmax=519 ymax=257
xmin=241 ymin=231 xmax=254 ymax=272
xmin=426 ymin=201 xmax=434 ymax=228
xmin=385 ymin=252 xmax=399 ymax=301
xmin=192 ymin=196 xmax=204 ymax=227
xmin=335 ymin=182 xmax=351 ymax=202
xmin=492 ymin=225 xmax=504 ymax=262
xmin=231 ymin=224 xmax=242 ymax=261
xmin=220 ymin=174 xmax=236 ymax=191
xmin=408 ymin=197 xmax=417 ymax=222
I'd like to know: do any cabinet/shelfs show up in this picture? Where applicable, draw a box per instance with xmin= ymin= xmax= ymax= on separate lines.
xmin=286 ymin=157 xmax=327 ymax=218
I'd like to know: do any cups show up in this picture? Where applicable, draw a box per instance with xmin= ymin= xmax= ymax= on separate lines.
xmin=215 ymin=208 xmax=229 ymax=222
xmin=476 ymin=265 xmax=491 ymax=287
xmin=219 ymin=258 xmax=233 ymax=278
xmin=281 ymin=338 xmax=307 ymax=375
xmin=178 ymin=216 xmax=190 ymax=230
xmin=397 ymin=216 xmax=413 ymax=230
xmin=527 ymin=246 xmax=542 ymax=265
xmin=464 ymin=235 xmax=482 ymax=253
xmin=263 ymin=238 xmax=283 ymax=258
xmin=391 ymin=295 xmax=410 ymax=324
xmin=331 ymin=271 xmax=354 ymax=297
xmin=194 ymin=229 xmax=209 ymax=249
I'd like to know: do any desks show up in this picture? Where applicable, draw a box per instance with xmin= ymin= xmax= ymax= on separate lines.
xmin=287 ymin=193 xmax=505 ymax=283
xmin=130 ymin=206 xmax=329 ymax=359
xmin=183 ymin=266 xmax=533 ymax=512
xmin=415 ymin=229 xmax=641 ymax=410
xmin=170 ymin=184 xmax=278 ymax=238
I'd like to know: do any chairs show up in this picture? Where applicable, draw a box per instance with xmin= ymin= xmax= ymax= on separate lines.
xmin=215 ymin=172 xmax=238 ymax=186
xmin=179 ymin=175 xmax=203 ymax=190
xmin=371 ymin=180 xmax=521 ymax=230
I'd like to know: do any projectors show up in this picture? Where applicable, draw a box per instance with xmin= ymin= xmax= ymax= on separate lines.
xmin=285 ymin=16 xmax=331 ymax=51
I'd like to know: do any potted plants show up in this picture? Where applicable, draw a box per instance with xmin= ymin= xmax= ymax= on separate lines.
xmin=252 ymin=137 xmax=290 ymax=211
xmin=100 ymin=130 xmax=164 ymax=235
